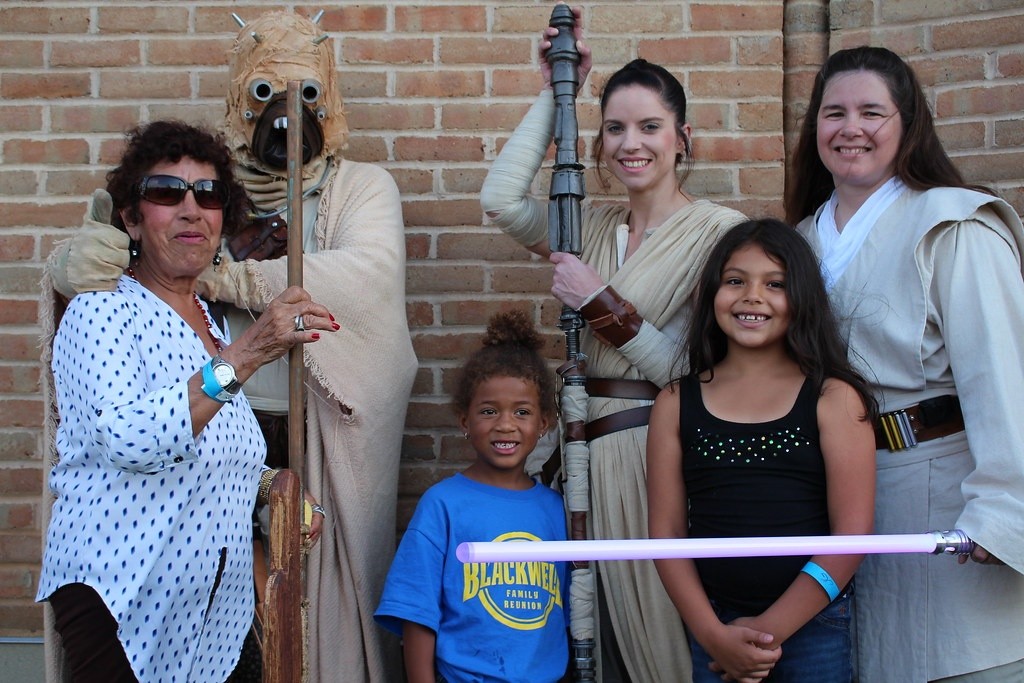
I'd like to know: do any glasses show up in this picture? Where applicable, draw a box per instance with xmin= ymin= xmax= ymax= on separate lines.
xmin=138 ymin=174 xmax=230 ymax=210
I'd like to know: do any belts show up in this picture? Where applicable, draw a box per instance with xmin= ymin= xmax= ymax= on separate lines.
xmin=251 ymin=408 xmax=307 ymax=471
xmin=540 ymin=378 xmax=661 ymax=487
xmin=871 ymin=394 xmax=965 ymax=453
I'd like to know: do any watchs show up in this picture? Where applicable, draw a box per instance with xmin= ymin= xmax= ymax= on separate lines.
xmin=211 ymin=354 xmax=242 ymax=395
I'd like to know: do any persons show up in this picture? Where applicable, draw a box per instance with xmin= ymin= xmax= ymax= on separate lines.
xmin=33 ymin=5 xmax=1024 ymax=683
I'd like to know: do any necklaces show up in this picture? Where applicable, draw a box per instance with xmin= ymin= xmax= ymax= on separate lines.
xmin=128 ymin=267 xmax=223 ymax=353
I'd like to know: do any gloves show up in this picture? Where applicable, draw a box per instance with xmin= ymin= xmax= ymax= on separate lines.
xmin=54 ymin=188 xmax=130 ymax=299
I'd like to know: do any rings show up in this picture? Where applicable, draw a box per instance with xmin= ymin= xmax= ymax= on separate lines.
xmin=294 ymin=316 xmax=299 ymax=328
xmin=297 ymin=317 xmax=304 ymax=330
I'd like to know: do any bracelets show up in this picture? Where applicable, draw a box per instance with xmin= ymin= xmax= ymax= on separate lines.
xmin=801 ymin=560 xmax=840 ymax=602
xmin=201 ymin=358 xmax=235 ymax=402
